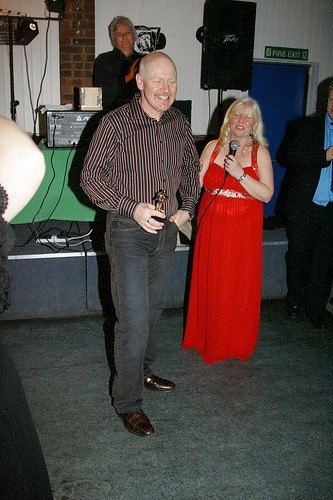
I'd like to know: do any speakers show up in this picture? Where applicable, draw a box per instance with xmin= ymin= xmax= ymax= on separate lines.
xmin=199 ymin=0 xmax=256 ymax=92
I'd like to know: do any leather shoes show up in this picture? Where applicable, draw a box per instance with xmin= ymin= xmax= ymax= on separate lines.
xmin=287 ymin=303 xmax=302 ymax=318
xmin=143 ymin=373 xmax=176 ymax=392
xmin=310 ymin=315 xmax=328 ymax=330
xmin=121 ymin=409 xmax=155 ymax=436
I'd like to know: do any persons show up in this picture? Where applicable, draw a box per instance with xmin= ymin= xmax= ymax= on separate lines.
xmin=278 ymin=79 xmax=333 ymax=328
xmin=185 ymin=96 xmax=274 ymax=366
xmin=94 ymin=14 xmax=143 ymax=110
xmin=0 ymin=115 xmax=54 ymax=500
xmin=81 ymin=52 xmax=201 ymax=437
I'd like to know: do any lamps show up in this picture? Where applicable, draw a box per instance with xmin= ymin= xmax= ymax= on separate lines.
xmin=15 ymin=16 xmax=39 ymax=46
xmin=44 ymin=0 xmax=65 ymax=18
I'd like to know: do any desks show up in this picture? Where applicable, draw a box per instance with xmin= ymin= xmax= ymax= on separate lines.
xmin=10 ymin=142 xmax=106 ymax=225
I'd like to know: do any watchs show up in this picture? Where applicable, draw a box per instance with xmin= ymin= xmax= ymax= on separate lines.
xmin=238 ymin=172 xmax=247 ymax=182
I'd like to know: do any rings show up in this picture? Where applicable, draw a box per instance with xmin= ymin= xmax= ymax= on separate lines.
xmin=147 ymin=217 xmax=151 ymax=223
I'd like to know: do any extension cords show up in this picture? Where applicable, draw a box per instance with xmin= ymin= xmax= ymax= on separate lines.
xmin=36 ymin=237 xmax=67 ymax=242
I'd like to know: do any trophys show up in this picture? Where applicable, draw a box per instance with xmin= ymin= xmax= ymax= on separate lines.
xmin=153 ymin=189 xmax=168 ymax=211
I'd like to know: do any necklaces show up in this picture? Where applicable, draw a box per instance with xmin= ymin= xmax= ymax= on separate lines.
xmin=232 ymin=136 xmax=252 ymax=156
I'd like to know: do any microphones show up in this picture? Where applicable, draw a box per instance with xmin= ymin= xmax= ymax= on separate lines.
xmin=225 ymin=140 xmax=240 ymax=177
